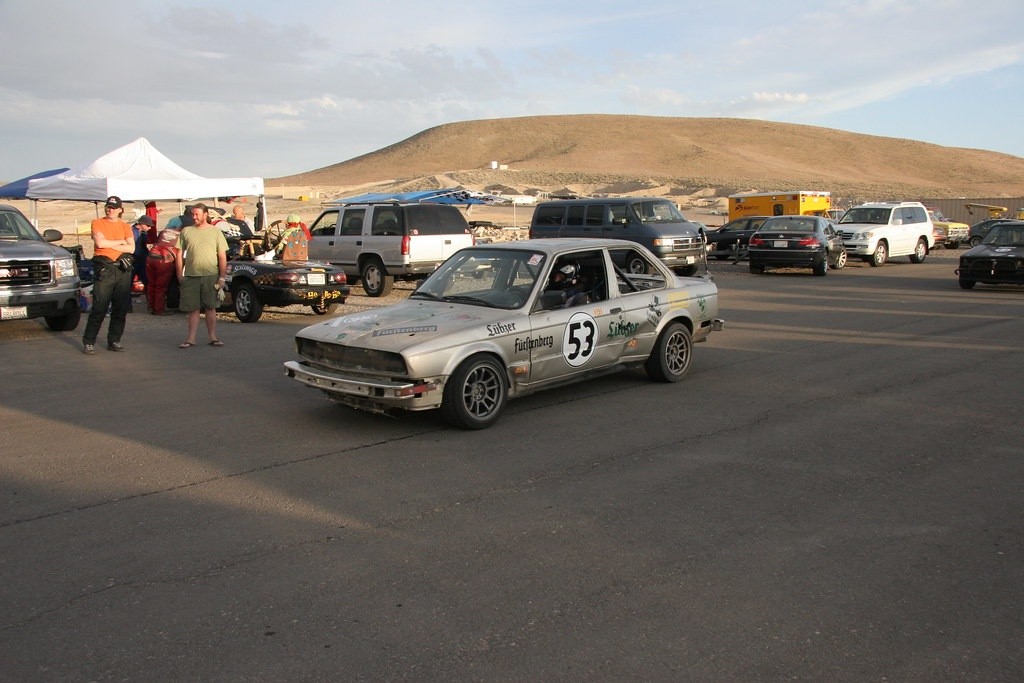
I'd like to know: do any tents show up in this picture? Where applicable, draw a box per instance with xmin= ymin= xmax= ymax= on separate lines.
xmin=0 ymin=168 xmax=98 ymax=224
xmin=26 ymin=137 xmax=270 ymax=245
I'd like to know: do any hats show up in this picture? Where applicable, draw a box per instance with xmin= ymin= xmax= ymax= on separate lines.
xmin=104 ymin=196 xmax=122 ymax=209
xmin=137 ymin=215 xmax=155 ymax=227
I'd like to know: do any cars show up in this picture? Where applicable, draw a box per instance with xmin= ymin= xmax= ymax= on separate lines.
xmin=166 ymin=235 xmax=352 ymax=323
xmin=689 ymin=220 xmax=709 ymax=234
xmin=703 ymin=215 xmax=775 ymax=260
xmin=967 ymin=219 xmax=1020 ymax=249
xmin=748 ymin=215 xmax=848 ymax=276
xmin=927 ymin=206 xmax=972 ymax=249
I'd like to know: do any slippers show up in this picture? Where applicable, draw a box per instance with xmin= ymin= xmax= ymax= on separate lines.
xmin=208 ymin=339 xmax=221 ymax=346
xmin=178 ymin=342 xmax=197 ymax=348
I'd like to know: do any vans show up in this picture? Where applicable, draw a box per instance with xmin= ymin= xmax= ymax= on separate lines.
xmin=834 ymin=200 xmax=935 ymax=267
xmin=528 ymin=196 xmax=706 ymax=278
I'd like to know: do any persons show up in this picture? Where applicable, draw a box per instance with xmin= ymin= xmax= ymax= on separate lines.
xmin=82 ymin=196 xmax=135 ymax=355
xmin=128 ymin=215 xmax=154 ymax=313
xmin=174 ymin=203 xmax=230 ymax=348
xmin=545 ymin=259 xmax=588 ymax=307
xmin=166 ymin=205 xmax=254 ymax=255
xmin=247 ymin=214 xmax=312 ymax=261
xmin=145 ymin=225 xmax=186 ymax=316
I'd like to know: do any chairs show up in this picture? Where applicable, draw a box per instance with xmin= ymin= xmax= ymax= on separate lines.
xmin=66 ymin=245 xmax=86 ymax=266
xmin=860 ymin=211 xmax=870 ymax=221
xmin=770 ymin=225 xmax=787 ymax=230
xmin=346 ymin=218 xmax=362 ymax=235
xmin=384 ymin=219 xmax=396 ymax=229
xmin=799 ymin=224 xmax=814 ymax=230
xmin=579 ymin=265 xmax=607 ymax=302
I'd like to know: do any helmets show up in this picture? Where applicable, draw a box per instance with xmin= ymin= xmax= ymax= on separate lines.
xmin=548 ymin=258 xmax=581 ymax=289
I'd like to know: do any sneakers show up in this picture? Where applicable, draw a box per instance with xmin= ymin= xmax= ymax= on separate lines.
xmin=83 ymin=343 xmax=95 ymax=355
xmin=107 ymin=341 xmax=125 ymax=352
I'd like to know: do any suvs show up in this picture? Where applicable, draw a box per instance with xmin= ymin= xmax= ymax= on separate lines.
xmin=953 ymin=221 xmax=1024 ymax=289
xmin=0 ymin=203 xmax=83 ymax=332
xmin=305 ymin=201 xmax=477 ymax=297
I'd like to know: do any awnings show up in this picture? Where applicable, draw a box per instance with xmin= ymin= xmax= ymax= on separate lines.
xmin=320 ymin=188 xmax=515 ymax=204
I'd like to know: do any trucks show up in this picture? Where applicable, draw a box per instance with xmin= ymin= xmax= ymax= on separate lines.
xmin=727 ymin=190 xmax=853 ymax=228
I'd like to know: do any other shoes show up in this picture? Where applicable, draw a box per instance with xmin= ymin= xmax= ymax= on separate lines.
xmin=154 ymin=311 xmax=173 ymax=317
xmin=127 ymin=305 xmax=133 ymax=313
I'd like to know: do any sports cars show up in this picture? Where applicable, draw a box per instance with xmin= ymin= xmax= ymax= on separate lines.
xmin=282 ymin=235 xmax=726 ymax=430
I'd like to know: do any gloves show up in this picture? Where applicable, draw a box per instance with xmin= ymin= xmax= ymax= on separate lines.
xmin=215 ymin=283 xmax=228 ymax=302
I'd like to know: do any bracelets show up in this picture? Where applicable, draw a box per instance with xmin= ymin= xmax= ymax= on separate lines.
xmin=219 ymin=278 xmax=225 ymax=281
